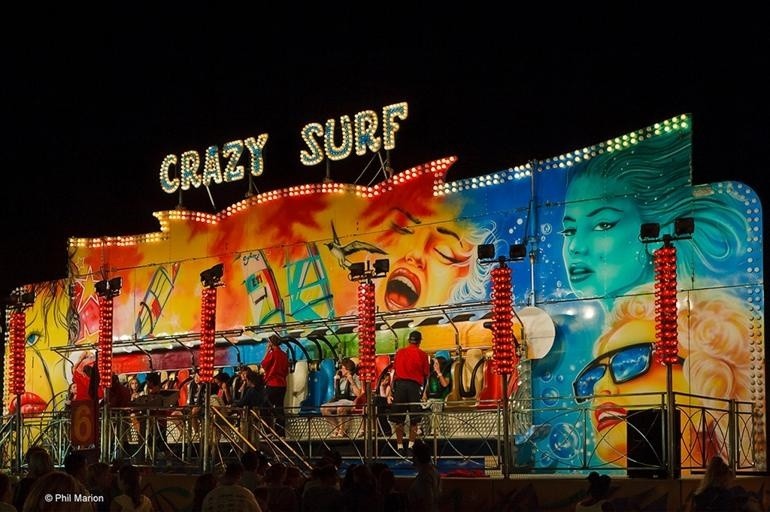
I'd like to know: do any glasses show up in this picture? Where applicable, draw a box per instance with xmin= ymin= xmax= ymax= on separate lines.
xmin=572 ymin=342 xmax=687 ymax=402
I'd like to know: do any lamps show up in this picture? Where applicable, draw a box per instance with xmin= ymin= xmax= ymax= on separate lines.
xmin=476 ymin=242 xmax=528 ymax=266
xmin=6 ymin=291 xmax=39 ymax=312
xmin=349 ymin=258 xmax=390 ymax=282
xmin=94 ymin=276 xmax=124 ymax=299
xmin=197 ymin=262 xmax=227 ymax=290
xmin=639 ymin=215 xmax=698 ymax=243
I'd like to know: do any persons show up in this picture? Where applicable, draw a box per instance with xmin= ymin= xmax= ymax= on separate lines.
xmin=376 ymin=190 xmax=499 ymax=313
xmin=3 ymin=283 xmax=80 ymax=452
xmin=505 ymin=454 xmax=770 ymax=512
xmin=573 ymin=279 xmax=766 ymax=474
xmin=561 ymin=129 xmax=745 ymax=315
xmin=0 ymin=329 xmax=452 ymax=511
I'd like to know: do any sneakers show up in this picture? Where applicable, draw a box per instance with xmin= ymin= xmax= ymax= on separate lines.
xmin=332 ymin=427 xmax=424 ymax=458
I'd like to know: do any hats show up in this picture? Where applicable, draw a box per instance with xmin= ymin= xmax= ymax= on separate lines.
xmin=341 ymin=359 xmax=355 ymax=371
xmin=434 ymin=351 xmax=449 ymax=361
xmin=409 ymin=330 xmax=422 ymax=342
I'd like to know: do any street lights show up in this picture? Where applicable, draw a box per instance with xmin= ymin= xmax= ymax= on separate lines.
xmin=92 ymin=275 xmax=123 ymax=464
xmin=196 ymin=260 xmax=226 ymax=470
xmin=5 ymin=291 xmax=37 ymax=472
xmin=637 ymin=217 xmax=700 ymax=473
xmin=346 ymin=258 xmax=391 ymax=467
xmin=472 ymin=241 xmax=528 ymax=478
